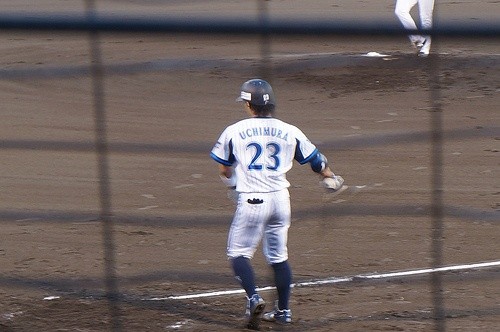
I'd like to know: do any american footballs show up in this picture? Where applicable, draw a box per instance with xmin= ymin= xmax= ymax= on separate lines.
xmin=321 ymin=175 xmax=345 ymax=191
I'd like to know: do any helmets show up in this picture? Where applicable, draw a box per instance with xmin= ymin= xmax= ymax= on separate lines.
xmin=235 ymin=79 xmax=275 ymax=105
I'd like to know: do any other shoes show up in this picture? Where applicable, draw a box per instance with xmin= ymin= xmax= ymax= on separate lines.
xmin=410 ymin=37 xmax=426 ymax=47
xmin=418 ymin=46 xmax=430 ymax=57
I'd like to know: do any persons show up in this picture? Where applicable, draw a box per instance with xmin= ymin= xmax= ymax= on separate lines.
xmin=209 ymin=79 xmax=344 ymax=328
xmin=395 ymin=0 xmax=436 ymax=56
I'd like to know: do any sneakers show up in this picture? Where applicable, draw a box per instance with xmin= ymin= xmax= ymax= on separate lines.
xmin=241 ymin=294 xmax=265 ymax=329
xmin=262 ymin=300 xmax=292 ymax=326
